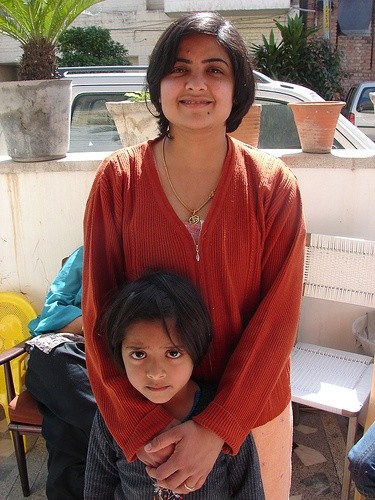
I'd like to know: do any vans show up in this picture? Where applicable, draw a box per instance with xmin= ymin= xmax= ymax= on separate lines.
xmin=340 ymin=80 xmax=375 ymax=144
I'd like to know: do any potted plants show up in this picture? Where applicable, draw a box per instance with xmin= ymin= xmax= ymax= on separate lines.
xmin=105 ymin=90 xmax=163 ymax=149
xmin=0 ymin=1 xmax=104 ymax=164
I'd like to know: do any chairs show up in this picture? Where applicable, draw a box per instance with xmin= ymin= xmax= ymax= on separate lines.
xmin=290 ymin=231 xmax=375 ymax=500
xmin=0 ymin=257 xmax=71 ymax=496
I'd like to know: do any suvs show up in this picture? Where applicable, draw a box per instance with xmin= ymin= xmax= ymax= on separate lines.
xmin=55 ymin=65 xmax=375 ymax=151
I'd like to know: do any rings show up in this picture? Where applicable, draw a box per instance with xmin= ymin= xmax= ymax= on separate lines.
xmin=184 ymin=481 xmax=194 ymax=491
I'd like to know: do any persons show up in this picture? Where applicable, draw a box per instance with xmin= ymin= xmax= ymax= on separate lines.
xmin=84 ymin=13 xmax=306 ymax=500
xmin=26 ymin=242 xmax=88 ymax=500
xmin=84 ymin=269 xmax=265 ymax=500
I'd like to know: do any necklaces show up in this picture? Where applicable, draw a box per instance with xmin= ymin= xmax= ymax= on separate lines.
xmin=162 ymin=132 xmax=219 ymax=225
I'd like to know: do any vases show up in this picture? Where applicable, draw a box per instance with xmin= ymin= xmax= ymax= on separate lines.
xmin=287 ymin=100 xmax=347 ymax=155
xmin=227 ymin=103 xmax=263 ymax=150
